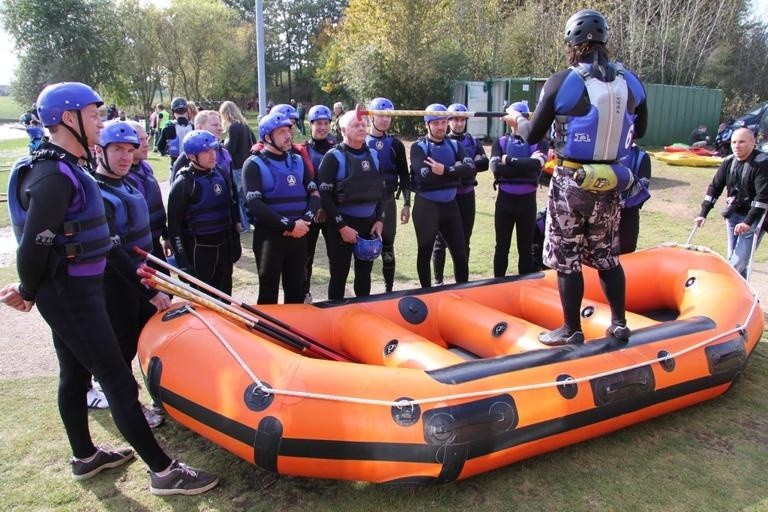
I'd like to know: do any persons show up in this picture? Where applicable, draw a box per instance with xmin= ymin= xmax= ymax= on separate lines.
xmin=503 ymin=7 xmax=649 ymax=347
xmin=715 ymin=121 xmax=732 ymax=150
xmin=0 ymin=80 xmax=222 ymax=497
xmin=88 ymin=120 xmax=174 ymax=428
xmin=250 ymin=102 xmax=323 ymax=304
xmin=687 ymin=123 xmax=710 ymax=149
xmin=620 ymin=141 xmax=652 ymax=252
xmin=696 ymin=126 xmax=768 ymax=279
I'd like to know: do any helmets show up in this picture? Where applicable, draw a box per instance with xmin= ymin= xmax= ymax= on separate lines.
xmin=352 ymin=231 xmax=386 ymax=264
xmin=33 ymin=80 xmax=107 ymax=129
xmin=182 ymin=128 xmax=220 ymax=153
xmin=505 ymin=101 xmax=530 ymax=113
xmin=268 ymin=103 xmax=299 ymax=120
xmin=563 ymin=7 xmax=610 ymax=48
xmin=170 ymin=96 xmax=188 ymax=112
xmin=257 ymin=111 xmax=293 ymax=144
xmin=423 ymin=102 xmax=449 ymax=123
xmin=447 ymin=103 xmax=467 ymax=113
xmin=95 ymin=117 xmax=141 ymax=150
xmin=369 ymin=97 xmax=395 ymax=112
xmin=308 ymin=104 xmax=332 ymax=124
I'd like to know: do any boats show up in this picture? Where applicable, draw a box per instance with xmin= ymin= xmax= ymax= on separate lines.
xmin=136 ymin=241 xmax=765 ymax=487
xmin=541 ymin=146 xmax=557 ymax=187
xmin=664 ymin=143 xmax=720 ymax=157
xmin=645 ymin=151 xmax=724 ymax=167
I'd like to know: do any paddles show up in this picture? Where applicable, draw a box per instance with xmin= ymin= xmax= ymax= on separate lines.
xmin=131 ymin=245 xmax=353 ymax=362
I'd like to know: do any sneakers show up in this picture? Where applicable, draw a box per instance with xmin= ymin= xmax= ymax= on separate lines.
xmin=68 ymin=440 xmax=135 ymax=480
xmin=146 ymin=458 xmax=221 ymax=496
xmin=188 ymin=484 xmax=190 ymax=486
xmin=604 ymin=325 xmax=632 ymax=343
xmin=86 ymin=384 xmax=110 ymax=409
xmin=137 ymin=402 xmax=166 ymax=429
xmin=536 ymin=327 xmax=584 ymax=347
